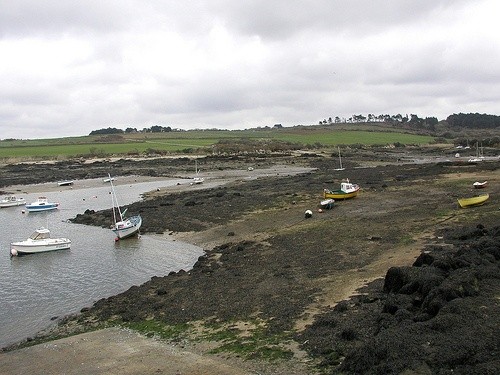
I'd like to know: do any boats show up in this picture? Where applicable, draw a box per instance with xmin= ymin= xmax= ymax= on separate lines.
xmin=457 ymin=191 xmax=489 ymax=209
xmin=472 ymin=181 xmax=488 ymax=189
xmin=0 ymin=195 xmax=26 ymax=208
xmin=323 ymin=178 xmax=360 ymax=200
xmin=58 ymin=181 xmax=74 ymax=186
xmin=320 ymin=198 xmax=335 ymax=210
xmin=103 ymin=178 xmax=117 ymax=183
xmin=9 ymin=226 xmax=72 ymax=254
xmin=25 ymin=196 xmax=60 ymax=213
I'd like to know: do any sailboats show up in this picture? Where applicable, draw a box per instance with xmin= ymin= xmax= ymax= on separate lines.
xmin=467 ymin=142 xmax=485 ymax=162
xmin=333 ymin=146 xmax=346 ymax=171
xmin=106 ymin=172 xmax=143 ymax=240
xmin=192 ymin=160 xmax=204 ymax=184
